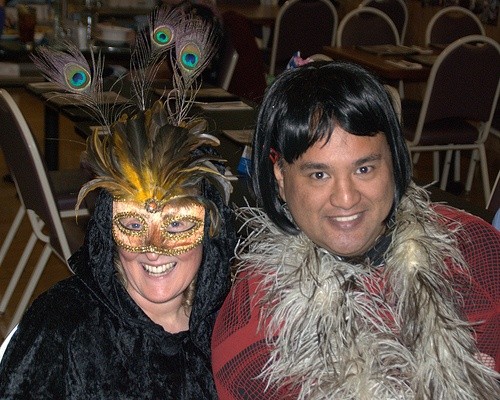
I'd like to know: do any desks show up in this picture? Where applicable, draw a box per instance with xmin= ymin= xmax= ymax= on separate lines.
xmin=235 ymin=6 xmax=276 ymax=26
xmin=322 ymin=45 xmax=440 ymax=78
xmin=30 ymin=80 xmax=261 ymax=174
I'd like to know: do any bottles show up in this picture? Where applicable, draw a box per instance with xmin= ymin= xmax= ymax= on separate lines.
xmin=73 ymin=12 xmax=89 ymax=52
xmin=85 ymin=0 xmax=98 ymax=39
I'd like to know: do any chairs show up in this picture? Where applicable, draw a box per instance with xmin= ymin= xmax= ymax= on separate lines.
xmin=222 ymin=50 xmax=239 ymax=89
xmin=270 ymin=0 xmax=500 ymax=208
xmin=0 ymin=90 xmax=89 ymax=348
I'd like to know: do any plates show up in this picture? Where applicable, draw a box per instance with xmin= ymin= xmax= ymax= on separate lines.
xmin=98 ymin=37 xmax=128 ymax=46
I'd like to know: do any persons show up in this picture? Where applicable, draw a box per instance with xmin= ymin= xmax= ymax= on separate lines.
xmin=211 ymin=56 xmax=500 ymax=400
xmin=0 ymin=6 xmax=244 ymax=400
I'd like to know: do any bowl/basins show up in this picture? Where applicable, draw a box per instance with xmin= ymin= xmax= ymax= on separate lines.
xmin=100 ymin=26 xmax=131 ymax=39
